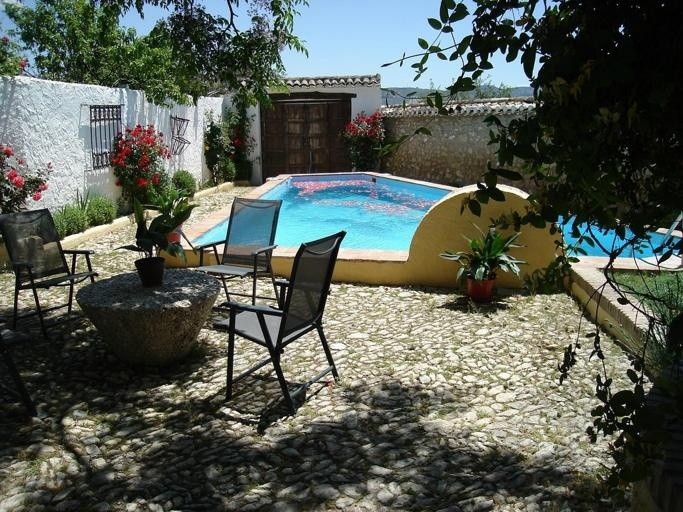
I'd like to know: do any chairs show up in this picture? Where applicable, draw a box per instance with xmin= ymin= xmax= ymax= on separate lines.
xmin=0 ymin=207 xmax=100 ymax=345
xmin=194 ymin=197 xmax=284 ymax=312
xmin=212 ymin=230 xmax=347 ymax=414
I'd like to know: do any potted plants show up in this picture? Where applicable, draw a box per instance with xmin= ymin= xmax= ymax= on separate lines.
xmin=112 ymin=197 xmax=202 ymax=288
xmin=440 ymin=226 xmax=526 ymax=302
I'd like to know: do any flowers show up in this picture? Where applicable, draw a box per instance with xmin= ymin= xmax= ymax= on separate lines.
xmin=109 ymin=126 xmax=172 ymax=207
xmin=0 ymin=144 xmax=52 ymax=214
xmin=340 ymin=111 xmax=386 ymax=172
